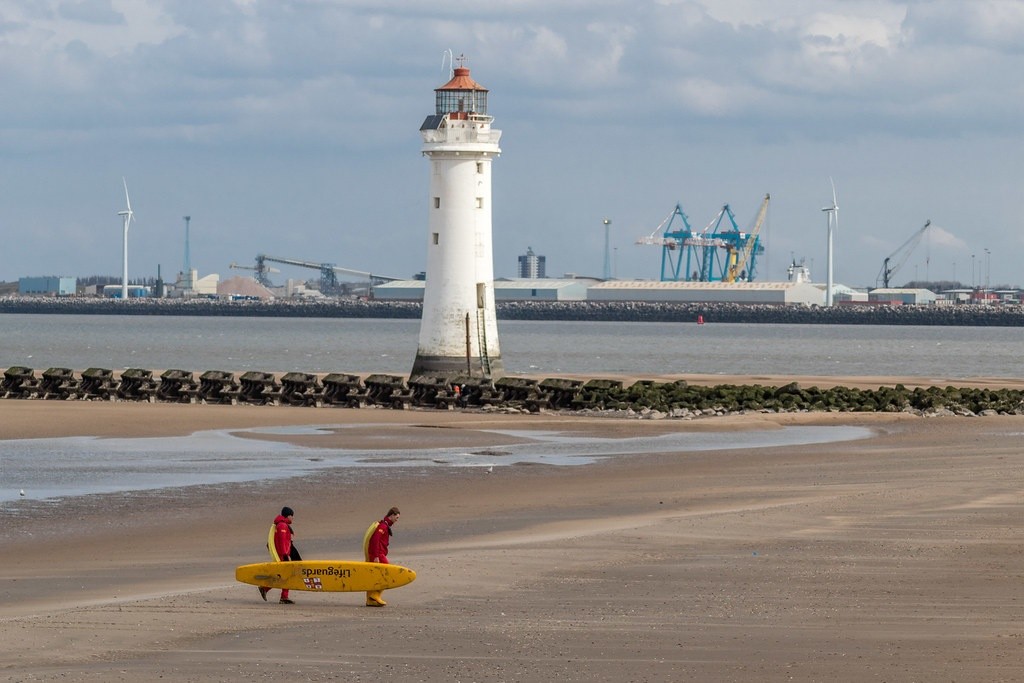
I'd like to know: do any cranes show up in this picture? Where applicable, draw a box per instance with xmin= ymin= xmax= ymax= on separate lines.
xmin=875 ymin=219 xmax=931 ymax=288
xmin=634 ymin=192 xmax=773 ymax=282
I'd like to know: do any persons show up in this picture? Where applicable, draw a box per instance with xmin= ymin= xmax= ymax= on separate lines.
xmin=365 ymin=507 xmax=400 ymax=606
xmin=259 ymin=506 xmax=295 ymax=605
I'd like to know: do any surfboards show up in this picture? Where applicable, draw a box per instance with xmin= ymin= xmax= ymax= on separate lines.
xmin=235 ymin=560 xmax=417 ymax=593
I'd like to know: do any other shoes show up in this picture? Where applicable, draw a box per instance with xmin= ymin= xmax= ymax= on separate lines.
xmin=279 ymin=598 xmax=295 ymax=604
xmin=258 ymin=586 xmax=267 ymax=601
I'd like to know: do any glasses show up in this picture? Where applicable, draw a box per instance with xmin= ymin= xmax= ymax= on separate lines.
xmin=291 ymin=514 xmax=293 ymax=516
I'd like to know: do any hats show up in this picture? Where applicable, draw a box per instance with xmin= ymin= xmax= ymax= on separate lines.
xmin=281 ymin=507 xmax=294 ymax=518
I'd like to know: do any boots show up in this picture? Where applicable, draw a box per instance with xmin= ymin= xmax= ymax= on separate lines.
xmin=369 ymin=589 xmax=386 ymax=605
xmin=366 ymin=591 xmax=384 ymax=607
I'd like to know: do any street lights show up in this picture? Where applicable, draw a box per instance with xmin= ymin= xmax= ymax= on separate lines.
xmin=971 ymin=248 xmax=991 ymax=289
